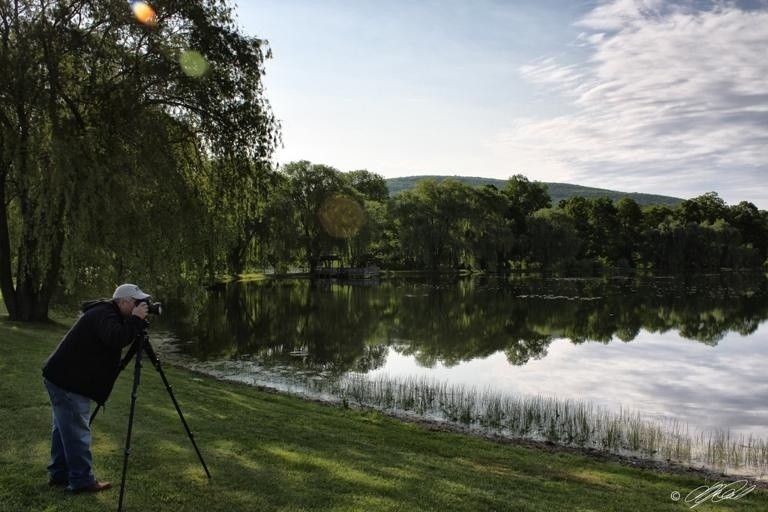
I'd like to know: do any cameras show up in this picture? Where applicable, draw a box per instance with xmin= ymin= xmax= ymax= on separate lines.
xmin=135 ymin=297 xmax=162 ymax=315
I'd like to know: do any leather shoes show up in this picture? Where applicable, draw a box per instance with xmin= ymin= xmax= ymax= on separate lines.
xmin=74 ymin=481 xmax=111 ymax=491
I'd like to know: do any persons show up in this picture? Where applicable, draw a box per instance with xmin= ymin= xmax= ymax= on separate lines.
xmin=40 ymin=283 xmax=154 ymax=494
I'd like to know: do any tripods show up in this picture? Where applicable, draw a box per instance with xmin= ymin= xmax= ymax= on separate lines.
xmin=89 ymin=336 xmax=211 ymax=512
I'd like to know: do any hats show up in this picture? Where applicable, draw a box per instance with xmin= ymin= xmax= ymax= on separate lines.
xmin=113 ymin=284 xmax=152 ymax=303
